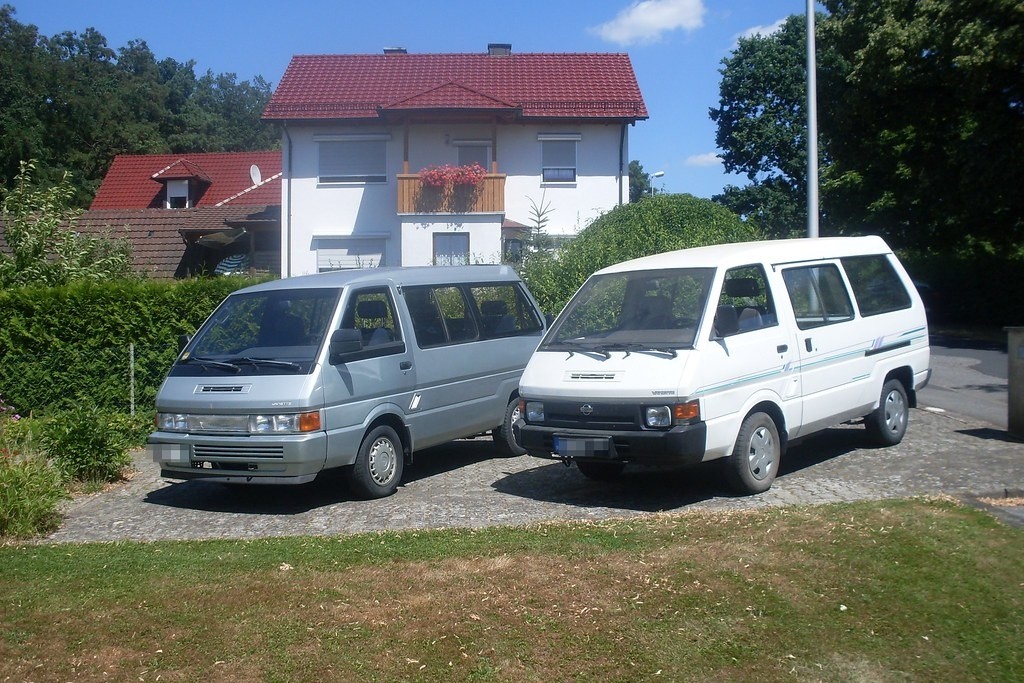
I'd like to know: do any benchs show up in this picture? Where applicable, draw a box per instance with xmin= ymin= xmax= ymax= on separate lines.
xmin=411 ymin=301 xmax=515 ymax=346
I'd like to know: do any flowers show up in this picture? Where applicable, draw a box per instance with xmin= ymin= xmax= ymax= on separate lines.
xmin=419 ymin=159 xmax=489 ymax=186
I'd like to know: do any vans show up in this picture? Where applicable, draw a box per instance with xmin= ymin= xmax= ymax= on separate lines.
xmin=511 ymin=234 xmax=932 ymax=497
xmin=145 ymin=262 xmax=547 ymax=501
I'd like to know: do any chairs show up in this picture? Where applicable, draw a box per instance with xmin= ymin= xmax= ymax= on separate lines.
xmin=259 ymin=311 xmax=304 ymax=343
xmin=620 ymin=296 xmax=673 ymax=328
xmin=726 ymin=277 xmax=770 ymax=329
xmin=357 ymin=301 xmax=393 ymax=345
